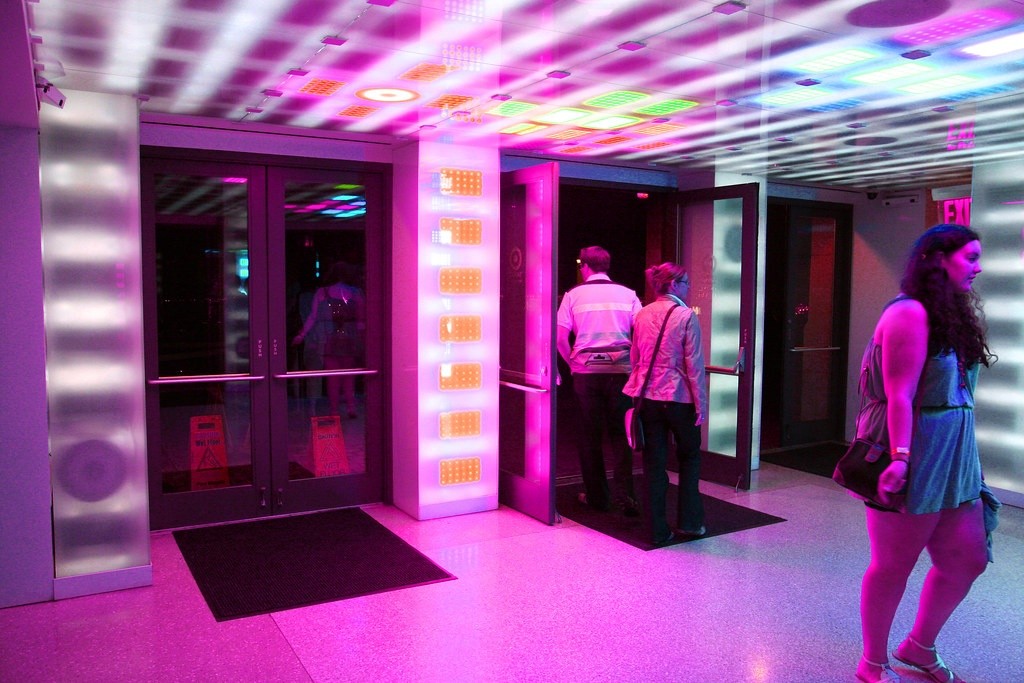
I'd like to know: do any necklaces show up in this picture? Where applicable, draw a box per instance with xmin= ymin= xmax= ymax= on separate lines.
xmin=957 ymin=355 xmax=968 ymax=388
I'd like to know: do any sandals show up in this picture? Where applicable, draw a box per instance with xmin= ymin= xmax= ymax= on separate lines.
xmin=855 ymin=653 xmax=904 ymax=683
xmin=892 ymin=633 xmax=967 ymax=683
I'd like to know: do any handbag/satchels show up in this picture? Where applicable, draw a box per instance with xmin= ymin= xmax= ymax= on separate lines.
xmin=624 ymin=407 xmax=645 ymax=451
xmin=832 ymin=438 xmax=913 ymax=511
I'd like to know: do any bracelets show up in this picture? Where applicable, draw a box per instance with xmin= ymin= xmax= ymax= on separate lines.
xmin=890 ymin=446 xmax=911 ymax=463
xmin=298 ymin=332 xmax=306 ymax=339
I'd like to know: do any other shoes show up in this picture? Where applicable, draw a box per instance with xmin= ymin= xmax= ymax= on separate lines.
xmin=678 ymin=525 xmax=707 ymax=537
xmin=578 ymin=492 xmax=612 ymax=511
xmin=616 ymin=498 xmax=640 ymax=516
xmin=653 ymin=531 xmax=675 ymax=546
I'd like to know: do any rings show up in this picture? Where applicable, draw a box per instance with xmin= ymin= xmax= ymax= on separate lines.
xmin=702 ymin=417 xmax=704 ymax=420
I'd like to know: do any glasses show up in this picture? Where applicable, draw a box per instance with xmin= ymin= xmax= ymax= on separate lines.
xmin=679 ymin=280 xmax=689 ymax=285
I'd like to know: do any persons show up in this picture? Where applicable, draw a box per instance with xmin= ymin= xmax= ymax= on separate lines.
xmin=293 ymin=263 xmax=371 ymax=419
xmin=844 ymin=223 xmax=998 ymax=683
xmin=557 ymin=245 xmax=643 ymax=516
xmin=622 ymin=262 xmax=707 ymax=549
